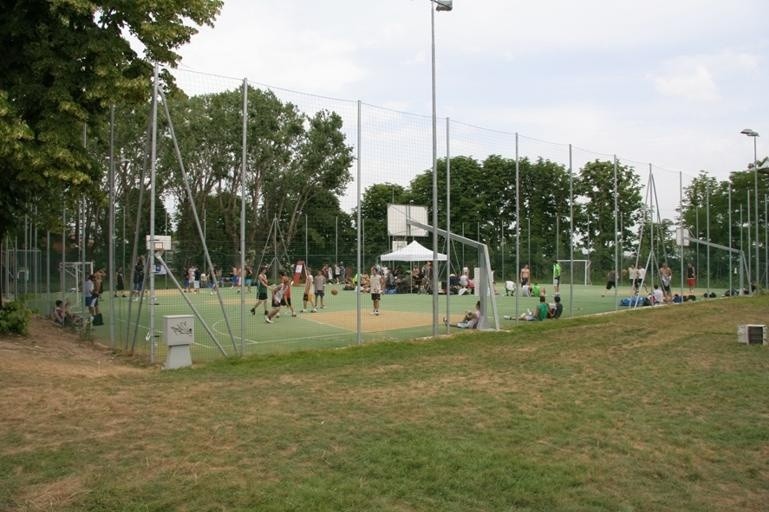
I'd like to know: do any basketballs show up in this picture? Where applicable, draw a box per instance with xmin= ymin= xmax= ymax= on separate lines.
xmin=331 ymin=287 xmax=338 ymax=295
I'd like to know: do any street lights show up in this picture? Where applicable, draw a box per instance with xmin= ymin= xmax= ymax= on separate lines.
xmin=385 ymin=181 xmax=396 ymax=205
xmin=676 ymin=208 xmax=682 ymax=213
xmin=741 ymin=126 xmax=762 ymax=288
xmin=109 ymin=156 xmax=134 ymax=169
xmin=429 ymin=0 xmax=453 ymax=338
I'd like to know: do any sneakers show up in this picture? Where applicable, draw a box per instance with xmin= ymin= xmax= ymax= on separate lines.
xmin=443 ymin=317 xmax=447 ymax=326
xmin=292 ymin=306 xmax=323 ymax=317
xmin=250 ymin=308 xmax=280 ymax=323
xmin=504 ymin=316 xmax=510 ymax=320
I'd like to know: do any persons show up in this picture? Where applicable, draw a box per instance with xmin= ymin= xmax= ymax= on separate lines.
xmin=345 ymin=262 xmax=475 ymax=295
xmin=369 ymin=268 xmax=384 ymax=314
xmin=83 ymin=271 xmax=106 ymax=327
xmin=183 ymin=266 xmax=252 ymax=293
xmin=129 ymin=255 xmax=145 ymax=298
xmin=600 ymin=260 xmax=757 ymax=308
xmin=53 ymin=299 xmax=80 ymax=326
xmin=506 ymin=258 xmax=565 ymax=322
xmin=113 ymin=265 xmax=127 ymax=297
xmin=443 ymin=300 xmax=481 ymax=330
xmin=250 ymin=261 xmax=348 ymax=323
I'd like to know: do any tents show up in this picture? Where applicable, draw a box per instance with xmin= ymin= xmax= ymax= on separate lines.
xmin=380 ymin=240 xmax=450 ymax=295
